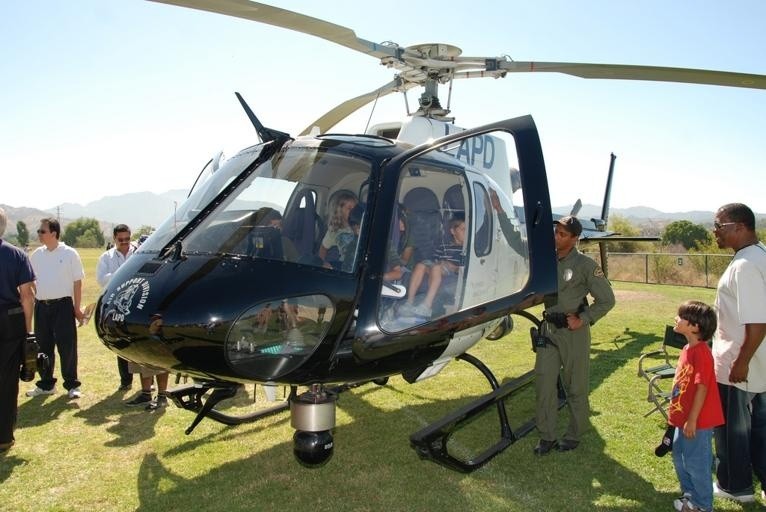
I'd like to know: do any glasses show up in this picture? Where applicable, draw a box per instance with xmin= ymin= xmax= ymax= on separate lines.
xmin=714 ymin=221 xmax=737 ymax=228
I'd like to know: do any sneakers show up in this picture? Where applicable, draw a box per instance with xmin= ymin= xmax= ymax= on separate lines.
xmin=119 ymin=384 xmax=132 ymax=390
xmin=150 ymin=384 xmax=156 ymax=391
xmin=395 ymin=301 xmax=432 ymax=318
xmin=143 ymin=393 xmax=169 ymax=412
xmin=26 ymin=383 xmax=57 ymax=396
xmin=712 ymin=480 xmax=754 ymax=502
xmin=124 ymin=390 xmax=153 ymax=407
xmin=68 ymin=385 xmax=80 ymax=399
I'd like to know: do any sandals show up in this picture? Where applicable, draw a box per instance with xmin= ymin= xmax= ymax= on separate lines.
xmin=674 ymin=494 xmax=712 ymax=511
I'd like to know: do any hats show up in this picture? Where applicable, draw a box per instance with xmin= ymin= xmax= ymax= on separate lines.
xmin=552 ymin=216 xmax=583 ymax=236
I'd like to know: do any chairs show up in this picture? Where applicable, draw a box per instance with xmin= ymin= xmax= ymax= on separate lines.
xmin=282 ymin=188 xmax=465 ymax=293
xmin=638 ymin=324 xmax=713 ymax=422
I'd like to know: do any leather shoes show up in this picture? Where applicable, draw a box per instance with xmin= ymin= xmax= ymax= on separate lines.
xmin=535 ymin=436 xmax=580 ymax=456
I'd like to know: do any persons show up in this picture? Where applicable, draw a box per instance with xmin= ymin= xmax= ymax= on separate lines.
xmin=667 ymin=300 xmax=727 ymax=512
xmin=95 ymin=224 xmax=157 ymax=391
xmin=711 ymin=202 xmax=766 ymax=504
xmin=24 ymin=217 xmax=86 ymax=399
xmin=248 ymin=189 xmax=467 ymax=320
xmin=487 ymin=187 xmax=616 ymax=456
xmin=125 ymin=236 xmax=169 ymax=412
xmin=1 ymin=205 xmax=38 ymax=451
xmin=96 ymin=296 xmax=301 ymax=347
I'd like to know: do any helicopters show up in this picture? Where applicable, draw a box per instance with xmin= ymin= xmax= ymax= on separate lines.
xmin=97 ymin=0 xmax=766 ymax=475
